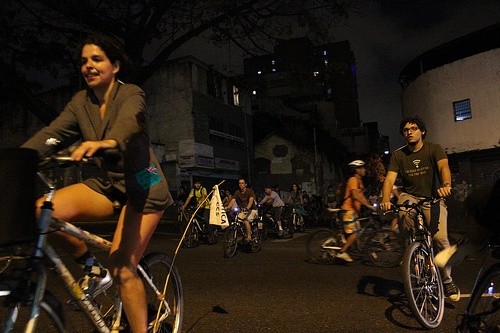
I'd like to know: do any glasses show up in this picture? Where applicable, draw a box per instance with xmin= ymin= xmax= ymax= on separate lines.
xmin=403 ymin=127 xmax=419 ymax=133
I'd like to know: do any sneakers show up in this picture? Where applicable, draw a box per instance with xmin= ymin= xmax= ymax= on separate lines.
xmin=441 ymin=277 xmax=461 ymax=302
xmin=65 ymin=268 xmax=114 ymax=310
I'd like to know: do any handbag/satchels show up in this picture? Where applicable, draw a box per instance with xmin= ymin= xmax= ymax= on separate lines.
xmin=335 ymin=198 xmax=345 ymax=208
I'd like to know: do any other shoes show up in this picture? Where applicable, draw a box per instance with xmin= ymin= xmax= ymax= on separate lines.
xmin=245 ymin=237 xmax=252 ymax=240
xmin=278 ymin=229 xmax=284 ymax=236
xmin=335 ymin=252 xmax=353 ymax=262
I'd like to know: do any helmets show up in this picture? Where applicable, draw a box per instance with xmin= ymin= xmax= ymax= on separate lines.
xmin=347 ymin=160 xmax=366 ymax=168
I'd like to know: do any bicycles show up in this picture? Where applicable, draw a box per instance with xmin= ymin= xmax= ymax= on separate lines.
xmin=178 ymin=199 xmax=342 ymax=258
xmin=304 ymin=207 xmax=407 ymax=269
xmin=382 ymin=194 xmax=447 ymax=328
xmin=0 ymin=154 xmax=183 ymax=333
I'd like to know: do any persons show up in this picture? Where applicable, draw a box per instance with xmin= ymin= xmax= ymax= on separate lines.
xmin=173 ymin=173 xmax=400 ymax=241
xmin=19 ymin=33 xmax=174 ymax=333
xmin=380 ymin=117 xmax=460 ymax=301
xmin=334 ymin=160 xmax=377 ymax=264
